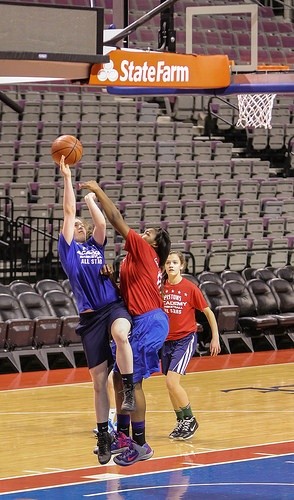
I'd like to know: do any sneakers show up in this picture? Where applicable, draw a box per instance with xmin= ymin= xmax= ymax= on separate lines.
xmin=97 ymin=433 xmax=111 ymax=464
xmin=113 ymin=439 xmax=153 ymax=466
xmin=121 ymin=392 xmax=135 ymax=411
xmin=169 ymin=416 xmax=199 ymax=441
xmin=94 ymin=432 xmax=132 ymax=454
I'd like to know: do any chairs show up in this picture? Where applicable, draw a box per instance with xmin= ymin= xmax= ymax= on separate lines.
xmin=0 ymin=0 xmax=294 ymax=374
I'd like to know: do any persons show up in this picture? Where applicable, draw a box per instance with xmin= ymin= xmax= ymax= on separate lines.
xmin=92 ymin=254 xmax=135 ymax=436
xmin=158 ymin=250 xmax=221 ymax=441
xmin=56 ymin=155 xmax=136 ymax=466
xmin=76 ymin=180 xmax=171 ymax=468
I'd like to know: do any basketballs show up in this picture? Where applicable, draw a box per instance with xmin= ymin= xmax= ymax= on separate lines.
xmin=52 ymin=135 xmax=83 ymax=165
